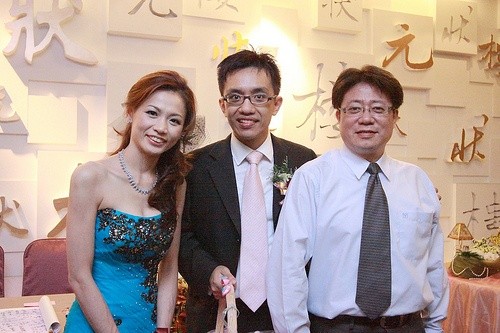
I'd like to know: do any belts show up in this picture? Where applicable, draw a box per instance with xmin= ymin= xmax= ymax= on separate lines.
xmin=337 ymin=311 xmax=417 ymax=329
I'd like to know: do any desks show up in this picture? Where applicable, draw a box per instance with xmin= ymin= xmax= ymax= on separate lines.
xmin=0 ymin=292 xmax=75 ymax=333
xmin=440 ymin=261 xmax=500 ymax=333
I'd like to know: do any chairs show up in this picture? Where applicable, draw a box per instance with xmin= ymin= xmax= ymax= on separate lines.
xmin=22 ymin=238 xmax=74 ymax=296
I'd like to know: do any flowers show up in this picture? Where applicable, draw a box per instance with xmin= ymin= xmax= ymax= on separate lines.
xmin=266 ymin=155 xmax=297 ymax=205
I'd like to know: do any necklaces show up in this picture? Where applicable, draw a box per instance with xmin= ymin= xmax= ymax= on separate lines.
xmin=118 ymin=148 xmax=159 ymax=195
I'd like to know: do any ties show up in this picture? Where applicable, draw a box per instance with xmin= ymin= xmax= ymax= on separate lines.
xmin=355 ymin=162 xmax=391 ymax=319
xmin=239 ymin=151 xmax=270 ymax=311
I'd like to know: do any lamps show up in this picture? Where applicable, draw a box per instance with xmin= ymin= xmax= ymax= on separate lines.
xmin=448 ymin=223 xmax=474 ymax=250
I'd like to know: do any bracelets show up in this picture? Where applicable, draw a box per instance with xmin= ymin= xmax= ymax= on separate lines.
xmin=155 ymin=326 xmax=177 ymax=333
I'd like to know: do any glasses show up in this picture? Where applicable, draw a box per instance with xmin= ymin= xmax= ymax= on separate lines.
xmin=223 ymin=93 xmax=277 ymax=106
xmin=338 ymin=106 xmax=397 ymax=116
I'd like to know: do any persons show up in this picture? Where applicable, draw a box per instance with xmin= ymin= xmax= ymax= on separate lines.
xmin=64 ymin=69 xmax=197 ymax=333
xmin=265 ymin=64 xmax=451 ymax=333
xmin=176 ymin=49 xmax=319 ymax=333
